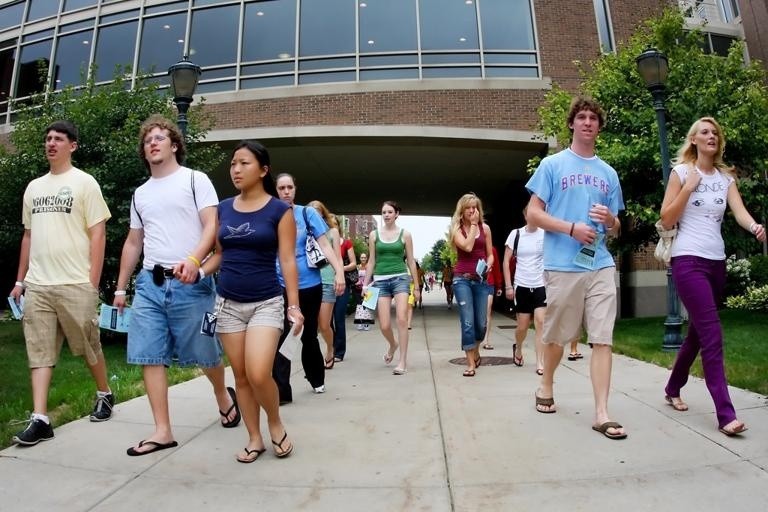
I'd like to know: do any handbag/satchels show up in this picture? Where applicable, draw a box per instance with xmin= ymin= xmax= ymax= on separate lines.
xmin=341 ymin=239 xmax=360 ymax=284
xmin=301 ymin=207 xmax=331 ymax=270
xmin=653 ymin=216 xmax=678 ymax=268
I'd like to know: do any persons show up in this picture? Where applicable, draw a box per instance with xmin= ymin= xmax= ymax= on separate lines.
xmin=307 ymin=200 xmax=343 ymax=369
xmin=525 ymin=96 xmax=628 ymax=440
xmin=503 ymin=209 xmax=547 ymax=375
xmin=355 ymin=193 xmax=502 ymax=376
xmin=272 ymin=172 xmax=346 ymax=406
xmin=568 ymin=337 xmax=583 ymax=361
xmin=173 ymin=141 xmax=304 ymax=463
xmin=660 ymin=117 xmax=767 ymax=435
xmin=330 ymin=216 xmax=357 ymax=362
xmin=9 ymin=120 xmax=112 ymax=445
xmin=113 ymin=113 xmax=240 ymax=456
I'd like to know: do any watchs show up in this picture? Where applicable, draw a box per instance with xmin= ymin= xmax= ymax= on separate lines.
xmin=607 ymin=219 xmax=616 ymax=231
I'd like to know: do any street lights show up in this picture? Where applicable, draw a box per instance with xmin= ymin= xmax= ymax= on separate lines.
xmin=635 ymin=46 xmax=687 ymax=350
xmin=166 ymin=55 xmax=201 ymax=140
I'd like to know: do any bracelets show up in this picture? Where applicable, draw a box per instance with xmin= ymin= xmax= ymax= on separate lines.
xmin=286 ymin=305 xmax=303 ymax=312
xmin=115 ymin=290 xmax=126 ymax=295
xmin=15 ymin=281 xmax=24 ymax=287
xmin=750 ymin=223 xmax=757 ymax=234
xmin=187 ymin=257 xmax=200 ymax=266
xmin=196 ymin=267 xmax=205 ymax=282
xmin=570 ymin=223 xmax=575 ymax=236
xmin=505 ymin=287 xmax=513 ymax=290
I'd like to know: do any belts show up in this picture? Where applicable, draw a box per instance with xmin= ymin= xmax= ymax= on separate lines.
xmin=150 ymin=266 xmax=178 ymax=280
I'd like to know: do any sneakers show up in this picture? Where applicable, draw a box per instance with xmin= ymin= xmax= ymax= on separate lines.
xmin=9 ymin=408 xmax=57 ymax=447
xmin=313 ymin=383 xmax=326 ymax=394
xmin=355 ymin=303 xmax=453 ymax=332
xmin=88 ymin=388 xmax=116 ymax=423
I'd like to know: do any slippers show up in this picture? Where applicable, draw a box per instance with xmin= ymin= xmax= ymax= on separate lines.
xmin=461 ymin=342 xmax=585 ymax=377
xmin=590 ymin=420 xmax=629 ymax=441
xmin=218 ymin=386 xmax=242 ymax=429
xmin=271 ymin=429 xmax=294 ymax=459
xmin=236 ymin=446 xmax=267 ymax=464
xmin=323 ymin=341 xmax=410 ymax=376
xmin=534 ymin=386 xmax=559 ymax=416
xmin=663 ymin=392 xmax=690 ymax=412
xmin=717 ymin=419 xmax=748 ymax=437
xmin=126 ymin=439 xmax=179 ymax=457
